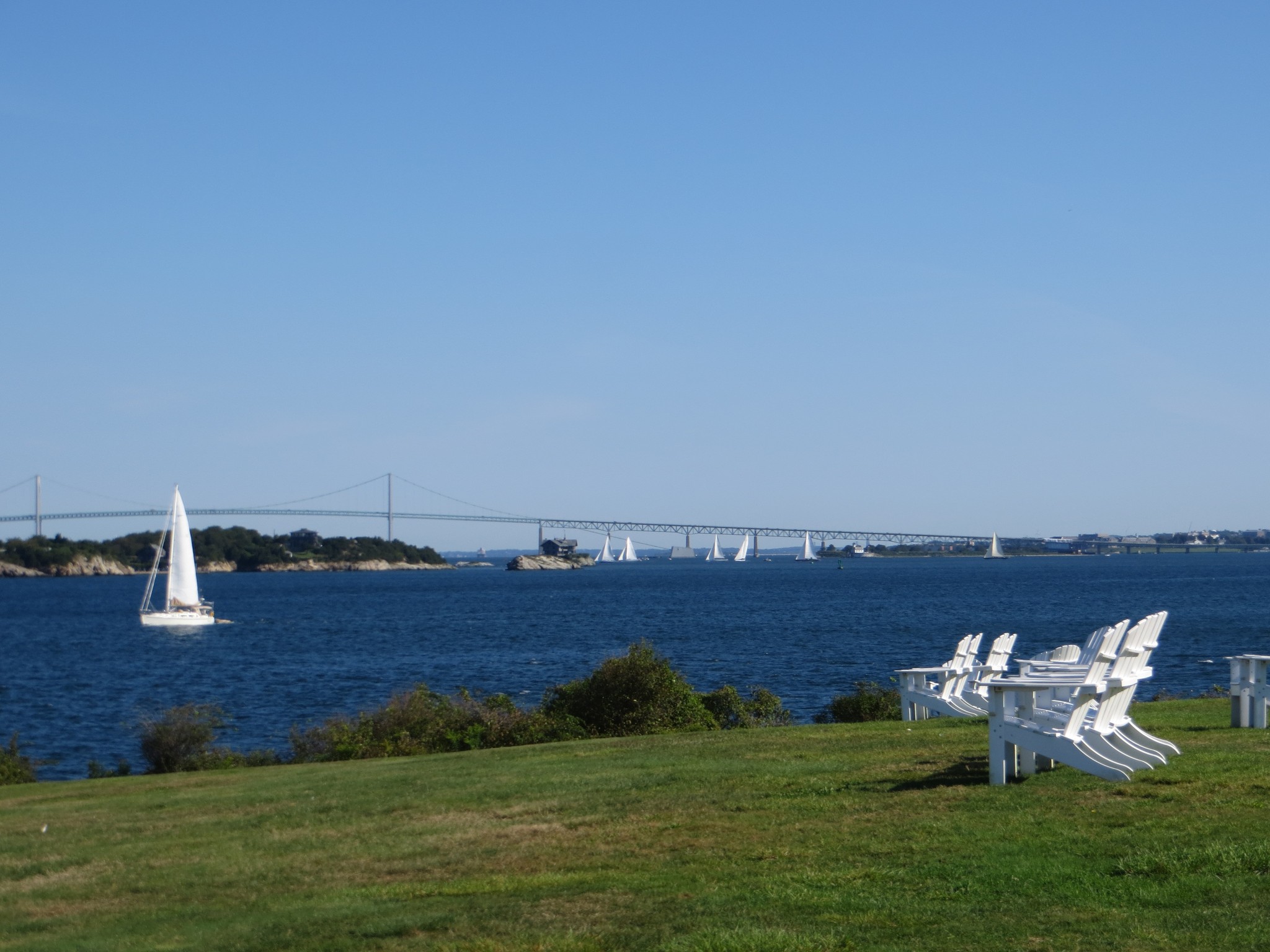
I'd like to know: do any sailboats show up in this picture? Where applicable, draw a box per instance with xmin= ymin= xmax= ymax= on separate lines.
xmin=594 ymin=535 xmax=615 ymax=562
xmin=795 ymin=531 xmax=819 ymax=561
xmin=984 ymin=531 xmax=1008 ymax=559
xmin=619 ymin=536 xmax=638 ymax=562
xmin=138 ymin=480 xmax=216 ymax=627
xmin=733 ymin=533 xmax=749 ymax=561
xmin=706 ymin=533 xmax=728 ymax=561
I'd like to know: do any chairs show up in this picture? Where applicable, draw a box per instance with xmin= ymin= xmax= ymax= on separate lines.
xmin=1225 ymin=650 xmax=1270 ymax=730
xmin=979 ymin=610 xmax=1183 ymax=787
xmin=894 ymin=632 xmax=1016 ymax=722
